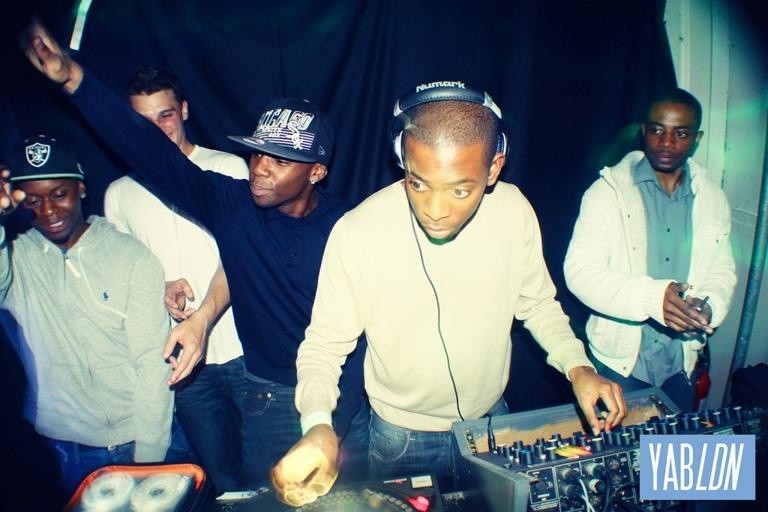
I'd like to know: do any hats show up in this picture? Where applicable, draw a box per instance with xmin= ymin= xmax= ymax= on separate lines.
xmin=227 ymin=98 xmax=335 ymax=167
xmin=10 ymin=135 xmax=85 ymax=181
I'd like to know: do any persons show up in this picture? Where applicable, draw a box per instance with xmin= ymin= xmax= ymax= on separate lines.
xmin=16 ymin=10 xmax=371 ymax=481
xmin=560 ymin=86 xmax=739 ymax=416
xmin=101 ymin=72 xmax=256 ymax=503
xmin=0 ymin=131 xmax=179 ymax=501
xmin=271 ymin=78 xmax=630 ymax=509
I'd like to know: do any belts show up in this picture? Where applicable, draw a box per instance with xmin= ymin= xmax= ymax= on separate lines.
xmin=62 ymin=441 xmax=135 ymax=451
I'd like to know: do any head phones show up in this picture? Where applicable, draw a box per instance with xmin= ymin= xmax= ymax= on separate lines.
xmin=387 ymin=81 xmax=510 ymax=171
